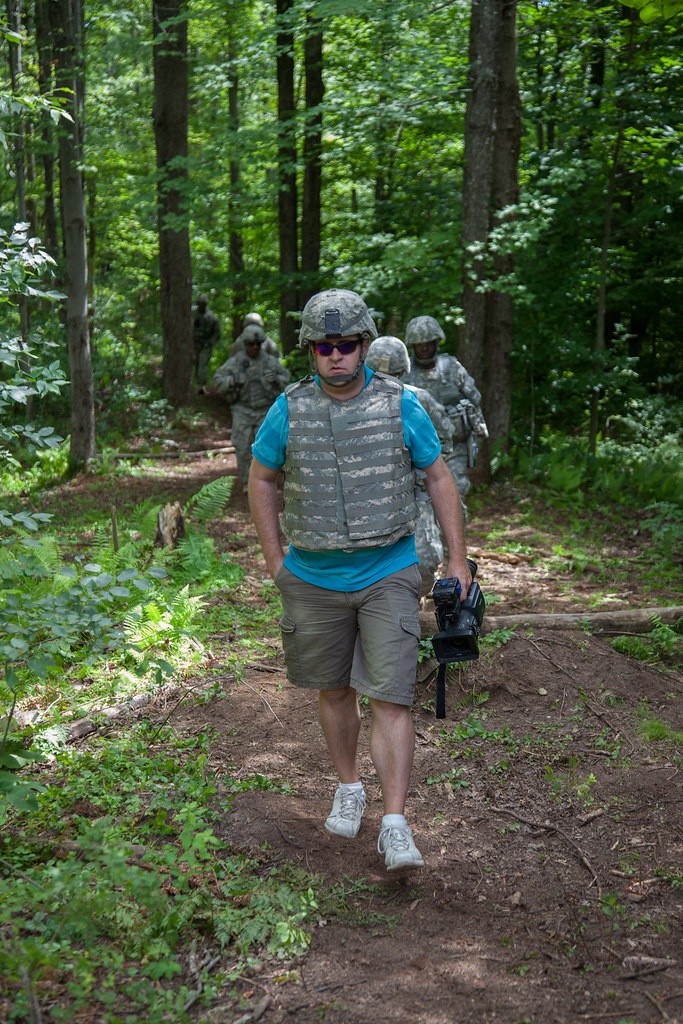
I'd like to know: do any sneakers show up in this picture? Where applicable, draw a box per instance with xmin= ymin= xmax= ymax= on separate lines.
xmin=325 ymin=788 xmax=368 ymax=838
xmin=380 ymin=822 xmax=426 ymax=872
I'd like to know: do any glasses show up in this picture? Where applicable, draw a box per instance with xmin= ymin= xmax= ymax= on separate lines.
xmin=311 ymin=339 xmax=361 ymax=356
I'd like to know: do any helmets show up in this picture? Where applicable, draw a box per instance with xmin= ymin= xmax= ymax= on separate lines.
xmin=298 ymin=290 xmax=377 ymax=350
xmin=195 ymin=294 xmax=210 ymax=304
xmin=241 ymin=325 xmax=267 ymax=343
xmin=365 ymin=336 xmax=411 ymax=374
xmin=244 ymin=313 xmax=264 ymax=330
xmin=405 ymin=315 xmax=445 ymax=347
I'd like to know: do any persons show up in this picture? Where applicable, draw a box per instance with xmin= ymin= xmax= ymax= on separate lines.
xmin=212 ymin=325 xmax=293 ymax=497
xmin=248 ymin=287 xmax=475 ymax=874
xmin=401 ymin=314 xmax=489 ymax=553
xmin=362 ymin=337 xmax=456 ymax=612
xmin=190 ymin=293 xmax=222 ymax=398
xmin=229 ymin=312 xmax=280 ymax=363
